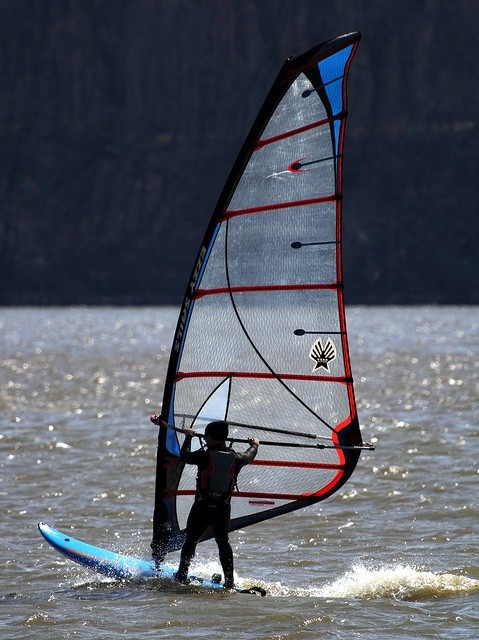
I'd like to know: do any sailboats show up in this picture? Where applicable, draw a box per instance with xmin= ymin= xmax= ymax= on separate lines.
xmin=38 ymin=28 xmax=376 ymax=598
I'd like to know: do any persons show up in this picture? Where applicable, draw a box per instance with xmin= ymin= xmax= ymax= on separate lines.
xmin=173 ymin=421 xmax=259 ymax=589
